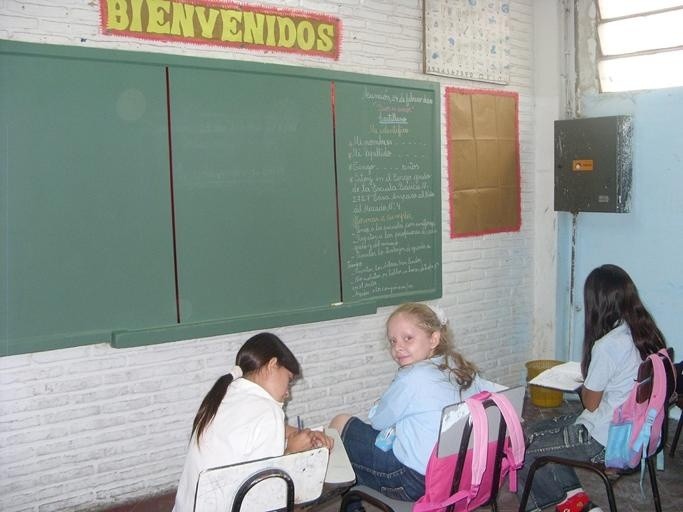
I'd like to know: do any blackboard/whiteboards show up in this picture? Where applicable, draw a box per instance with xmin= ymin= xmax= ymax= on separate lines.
xmin=0 ymin=38 xmax=442 ymax=357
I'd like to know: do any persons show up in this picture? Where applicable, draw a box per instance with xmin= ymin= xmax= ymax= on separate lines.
xmin=170 ymin=332 xmax=334 ymax=512
xmin=329 ymin=302 xmax=495 ymax=512
xmin=506 ymin=265 xmax=669 ymax=512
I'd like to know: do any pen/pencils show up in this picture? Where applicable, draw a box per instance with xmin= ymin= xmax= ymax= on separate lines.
xmin=297 ymin=415 xmax=301 ymax=433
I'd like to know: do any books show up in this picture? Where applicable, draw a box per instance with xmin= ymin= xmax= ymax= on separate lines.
xmin=527 ymin=359 xmax=584 ymax=393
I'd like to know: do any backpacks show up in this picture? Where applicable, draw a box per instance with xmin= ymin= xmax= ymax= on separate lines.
xmin=414 ymin=390 xmax=525 ymax=512
xmin=605 ymin=349 xmax=676 ymax=497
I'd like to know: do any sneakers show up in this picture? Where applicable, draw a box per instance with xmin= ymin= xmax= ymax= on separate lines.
xmin=555 ymin=493 xmax=603 ymax=512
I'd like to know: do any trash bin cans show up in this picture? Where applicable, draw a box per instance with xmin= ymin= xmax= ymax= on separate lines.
xmin=526 ymin=360 xmax=567 ymax=407
